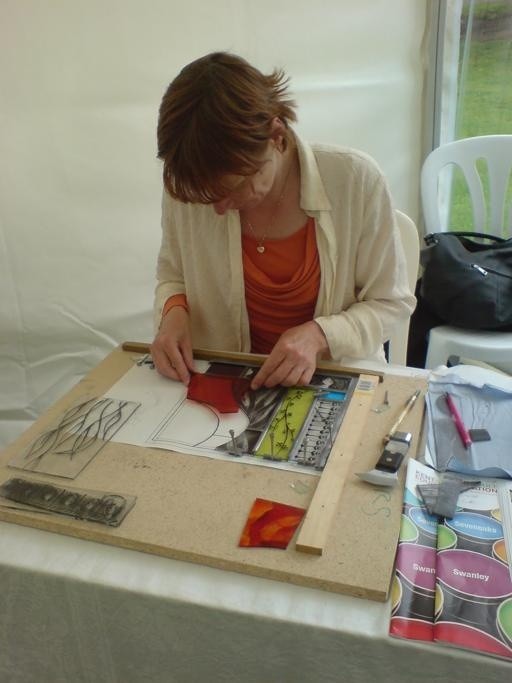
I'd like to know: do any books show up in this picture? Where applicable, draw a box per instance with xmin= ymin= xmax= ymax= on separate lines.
xmin=495 ymin=478 xmax=512 ymax=580
xmin=389 ymin=456 xmax=435 ymax=642
xmin=432 ymin=469 xmax=512 ymax=663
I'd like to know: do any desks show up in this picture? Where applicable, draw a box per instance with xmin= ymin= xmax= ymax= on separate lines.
xmin=0 ymin=519 xmax=512 ymax=683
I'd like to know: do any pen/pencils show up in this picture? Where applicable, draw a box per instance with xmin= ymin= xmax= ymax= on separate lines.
xmin=382 ymin=390 xmax=420 ymax=445
xmin=446 ymin=392 xmax=472 ymax=448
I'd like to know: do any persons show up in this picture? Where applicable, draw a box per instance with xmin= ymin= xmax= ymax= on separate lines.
xmin=152 ymin=52 xmax=419 ymax=394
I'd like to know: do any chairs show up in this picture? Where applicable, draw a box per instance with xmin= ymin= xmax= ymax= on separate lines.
xmin=420 ymin=133 xmax=512 ymax=375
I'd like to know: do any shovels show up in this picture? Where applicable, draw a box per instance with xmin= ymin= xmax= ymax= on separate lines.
xmin=353 ymin=430 xmax=412 ymax=490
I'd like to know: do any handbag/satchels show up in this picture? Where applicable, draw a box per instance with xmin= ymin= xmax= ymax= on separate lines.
xmin=417 ymin=230 xmax=512 ymax=332
